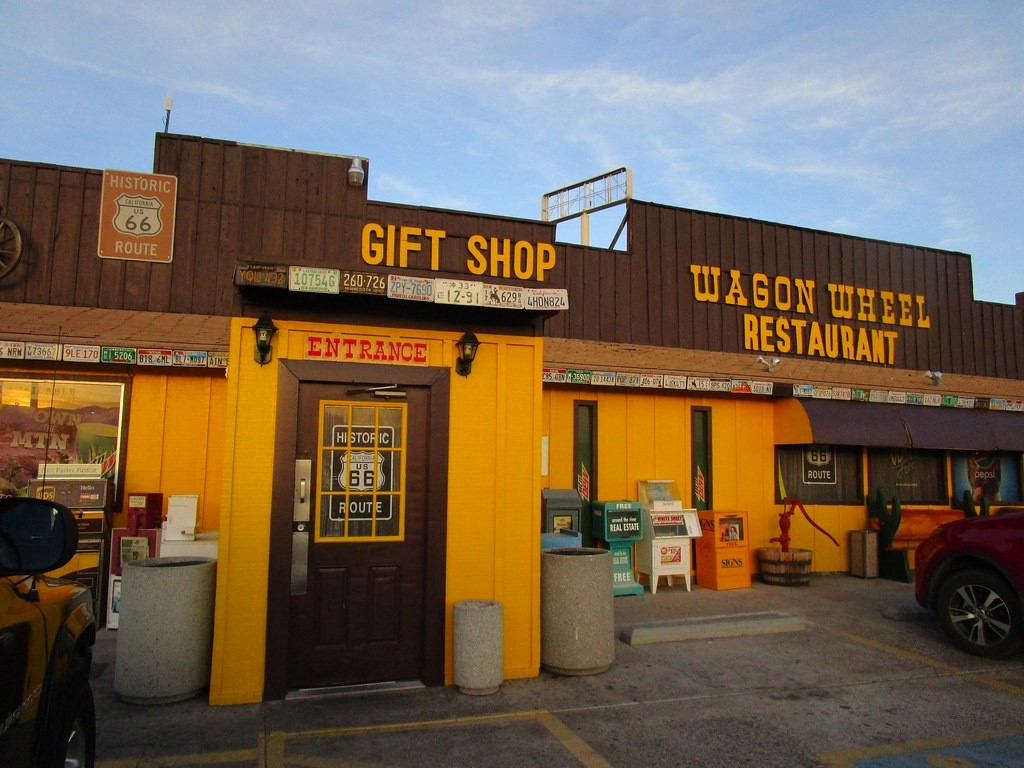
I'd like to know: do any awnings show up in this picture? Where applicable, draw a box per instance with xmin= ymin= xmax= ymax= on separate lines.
xmin=773 ymin=397 xmax=1024 ymax=452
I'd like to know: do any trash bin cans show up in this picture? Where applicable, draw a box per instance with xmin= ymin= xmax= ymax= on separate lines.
xmin=543 ymin=488 xmax=583 ymax=534
xmin=849 ymin=529 xmax=878 ymax=577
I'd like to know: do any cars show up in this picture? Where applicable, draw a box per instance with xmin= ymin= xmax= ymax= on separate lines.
xmin=0 ymin=498 xmax=99 ymax=768
xmin=913 ymin=511 xmax=1024 ymax=658
xmin=45 ymin=505 xmax=104 ymax=606
